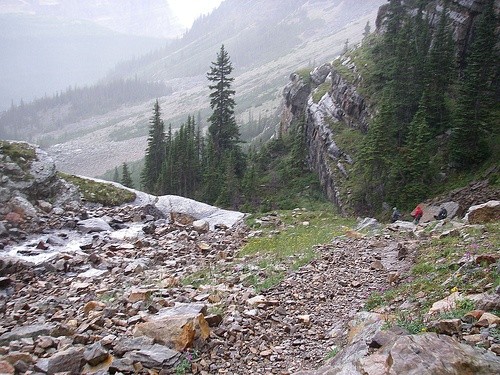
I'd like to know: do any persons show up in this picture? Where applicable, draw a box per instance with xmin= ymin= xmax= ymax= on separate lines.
xmin=411 ymin=206 xmax=423 ymax=223
xmin=391 ymin=207 xmax=399 ymax=222
xmin=436 ymin=205 xmax=448 ymax=219
